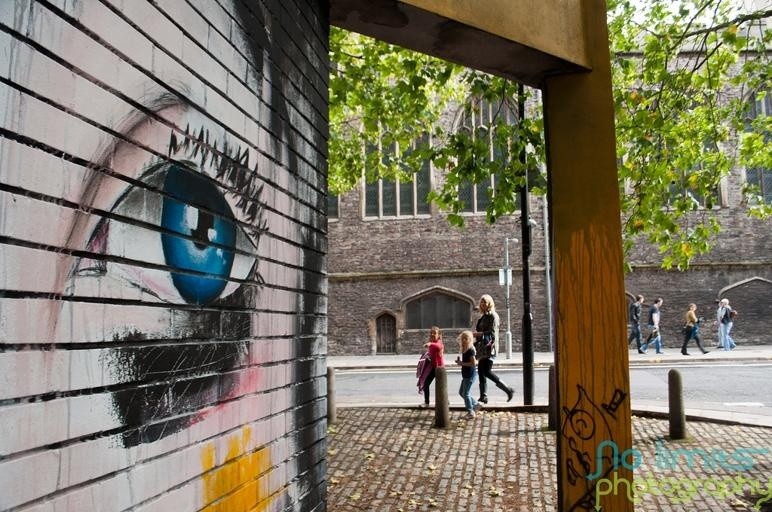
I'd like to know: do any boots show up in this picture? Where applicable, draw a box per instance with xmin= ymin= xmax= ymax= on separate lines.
xmin=496 ymin=378 xmax=513 ymax=400
xmin=478 ymin=383 xmax=488 ymax=404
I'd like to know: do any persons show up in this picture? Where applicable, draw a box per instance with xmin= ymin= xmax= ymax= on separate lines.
xmin=716 ymin=302 xmax=723 ymax=348
xmin=454 ymin=330 xmax=483 ymax=420
xmin=420 ymin=326 xmax=445 ymax=409
xmin=719 ymin=298 xmax=737 ymax=350
xmin=679 ymin=303 xmax=713 ymax=355
xmin=639 ymin=298 xmax=666 ymax=354
xmin=471 ymin=294 xmax=516 ymax=402
xmin=628 ymin=295 xmax=645 ymax=353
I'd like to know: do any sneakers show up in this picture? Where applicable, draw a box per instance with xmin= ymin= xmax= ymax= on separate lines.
xmin=462 ymin=411 xmax=475 ymax=419
xmin=474 ymin=402 xmax=482 ymax=412
xmin=420 ymin=404 xmax=427 ymax=408
xmin=639 ymin=347 xmax=665 ymax=354
xmin=681 ymin=350 xmax=709 ymax=354
xmin=718 ymin=344 xmax=736 ymax=350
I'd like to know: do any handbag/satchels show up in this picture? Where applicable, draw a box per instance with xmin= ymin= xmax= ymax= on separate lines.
xmin=730 ymin=311 xmax=737 ymax=319
xmin=682 ymin=326 xmax=687 ymax=335
xmin=474 ymin=334 xmax=496 ymax=361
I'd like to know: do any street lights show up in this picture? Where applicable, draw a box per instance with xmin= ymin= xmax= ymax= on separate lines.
xmin=502 ymin=236 xmax=518 ymax=358
